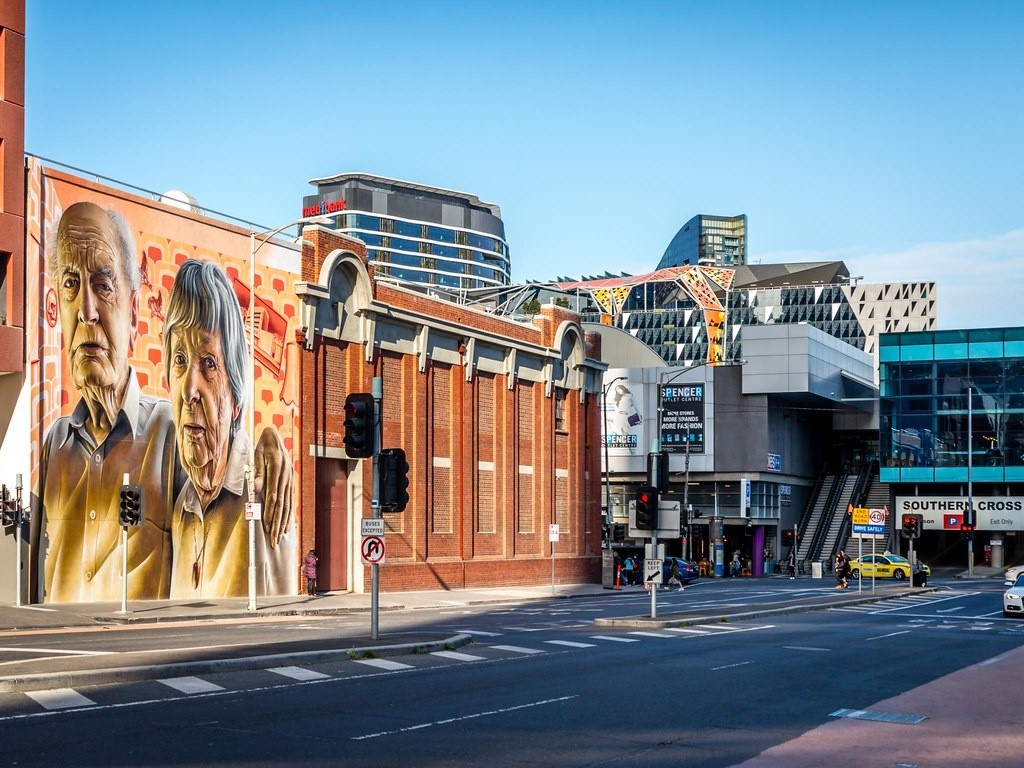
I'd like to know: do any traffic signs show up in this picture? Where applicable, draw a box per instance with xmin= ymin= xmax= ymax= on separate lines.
xmin=644 ymin=558 xmax=663 ymax=584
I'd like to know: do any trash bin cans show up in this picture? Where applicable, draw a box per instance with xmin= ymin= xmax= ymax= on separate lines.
xmin=812 ymin=560 xmax=824 ymax=579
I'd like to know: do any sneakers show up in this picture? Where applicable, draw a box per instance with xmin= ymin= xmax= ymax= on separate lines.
xmin=679 ymin=588 xmax=684 ymax=591
xmin=664 ymin=587 xmax=669 ymax=590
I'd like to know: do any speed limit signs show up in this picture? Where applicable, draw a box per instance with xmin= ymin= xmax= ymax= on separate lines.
xmin=870 ymin=511 xmax=882 ymax=523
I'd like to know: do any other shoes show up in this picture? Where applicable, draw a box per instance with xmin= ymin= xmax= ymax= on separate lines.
xmin=845 ymin=582 xmax=848 ymax=588
xmin=835 ymin=586 xmax=841 ymax=588
xmin=790 ymin=577 xmax=795 ymax=579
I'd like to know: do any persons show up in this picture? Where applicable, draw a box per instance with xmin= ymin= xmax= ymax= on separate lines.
xmin=664 ymin=558 xmax=685 ymax=592
xmin=613 ymin=551 xmax=627 ymax=585
xmin=613 ymin=384 xmax=642 ymax=455
xmin=305 ymin=549 xmax=317 ymax=598
xmin=728 ymin=549 xmax=742 ymax=578
xmin=37 ymin=201 xmax=298 ymax=601
xmin=788 ymin=551 xmax=795 ymax=579
xmin=634 ymin=553 xmax=642 ymax=585
xmin=624 ymin=554 xmax=635 ymax=586
xmin=834 ymin=549 xmax=849 ymax=589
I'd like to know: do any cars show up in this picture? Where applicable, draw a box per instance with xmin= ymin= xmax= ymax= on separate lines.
xmin=847 ymin=550 xmax=931 ymax=580
xmin=624 ymin=556 xmax=700 ymax=585
xmin=1003 ymin=565 xmax=1024 ymax=614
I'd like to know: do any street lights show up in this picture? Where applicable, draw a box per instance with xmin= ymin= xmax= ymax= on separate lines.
xmin=247 ymin=217 xmax=335 ymax=612
xmin=657 ymin=406 xmax=691 ymax=560
xmin=657 ymin=359 xmax=749 ymax=453
xmin=603 ymin=376 xmax=629 ymax=551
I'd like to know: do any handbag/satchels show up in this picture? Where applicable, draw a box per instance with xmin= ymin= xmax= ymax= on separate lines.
xmin=633 ymin=563 xmax=638 ymax=568
xmin=734 ymin=564 xmax=739 ymax=568
xmin=668 ymin=578 xmax=679 ymax=584
xmin=845 ymin=565 xmax=850 ymax=571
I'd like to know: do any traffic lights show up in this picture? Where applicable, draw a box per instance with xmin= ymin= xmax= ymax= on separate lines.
xmin=342 ymin=392 xmax=376 ymax=459
xmin=961 ymin=525 xmax=973 ymax=539
xmin=636 ymin=487 xmax=658 ymax=530
xmin=380 ymin=448 xmax=411 ymax=512
xmin=902 ymin=514 xmax=922 ymax=539
xmin=614 ymin=524 xmax=625 ymax=541
xmin=694 ymin=509 xmax=703 ymax=518
xmin=118 ymin=485 xmax=145 ymax=527
xmin=680 ymin=508 xmax=688 ymax=526
xmin=781 ymin=529 xmax=795 ymax=538
xmin=0 ymin=484 xmax=17 ymax=527
xmin=797 ymin=534 xmax=802 ymax=540
